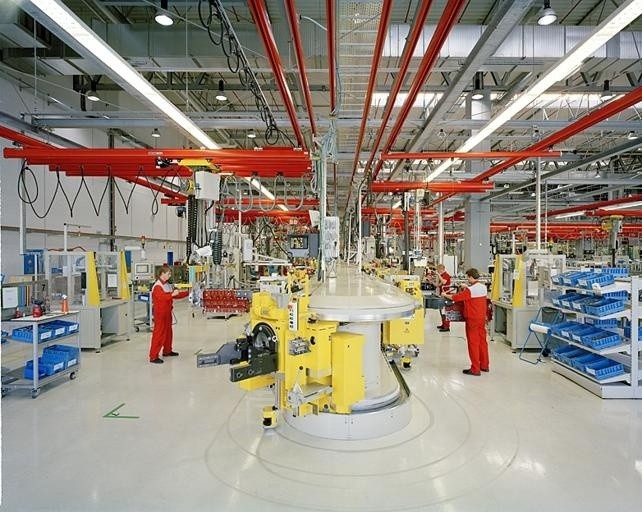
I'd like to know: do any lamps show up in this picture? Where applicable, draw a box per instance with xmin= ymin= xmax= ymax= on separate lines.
xmin=438 ymin=129 xmax=446 ymax=136
xmin=538 ymin=0 xmax=556 ymax=26
xmin=154 ymin=0 xmax=173 ymax=26
xmin=595 ymin=168 xmax=600 ymax=178
xmin=504 ymin=183 xmax=509 ymax=188
xmin=628 ymin=131 xmax=638 ymax=139
xmin=151 ymin=128 xmax=160 ymax=137
xmin=533 ymin=130 xmax=540 ymax=138
xmin=247 ymin=129 xmax=255 ymax=137
xmin=600 ymin=78 xmax=614 ymax=100
xmin=215 ymin=81 xmax=226 ymax=101
xmin=88 ymin=80 xmax=99 ymax=101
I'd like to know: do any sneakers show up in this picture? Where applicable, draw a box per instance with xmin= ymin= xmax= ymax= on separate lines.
xmin=437 ymin=326 xmax=447 ymax=329
xmin=439 ymin=328 xmax=452 ymax=333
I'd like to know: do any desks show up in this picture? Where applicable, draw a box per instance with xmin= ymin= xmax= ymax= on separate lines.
xmin=489 ymin=295 xmax=553 ymax=352
xmin=53 ymin=299 xmax=133 ymax=353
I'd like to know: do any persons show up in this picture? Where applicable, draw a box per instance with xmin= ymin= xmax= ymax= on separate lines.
xmin=441 ymin=268 xmax=490 ymax=376
xmin=149 ymin=266 xmax=179 ymax=364
xmin=429 ymin=264 xmax=451 ymax=332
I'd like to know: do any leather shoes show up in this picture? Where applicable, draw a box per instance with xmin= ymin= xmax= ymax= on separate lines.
xmin=480 ymin=368 xmax=490 ymax=372
xmin=150 ymin=358 xmax=164 ymax=363
xmin=462 ymin=369 xmax=481 ymax=377
xmin=163 ymin=352 xmax=178 ymax=356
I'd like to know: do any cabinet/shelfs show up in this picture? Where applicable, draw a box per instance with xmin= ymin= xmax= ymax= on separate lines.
xmin=2 ymin=307 xmax=81 ymax=397
xmin=550 ymin=268 xmax=632 ymax=400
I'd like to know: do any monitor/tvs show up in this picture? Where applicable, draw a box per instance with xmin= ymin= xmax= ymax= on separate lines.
xmin=135 ymin=264 xmax=149 ymax=274
xmin=107 ymin=273 xmax=118 ymax=288
xmin=1 ymin=286 xmax=18 ymax=309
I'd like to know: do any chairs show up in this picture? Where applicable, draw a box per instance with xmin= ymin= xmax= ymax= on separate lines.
xmin=521 ymin=307 xmax=563 ymax=363
